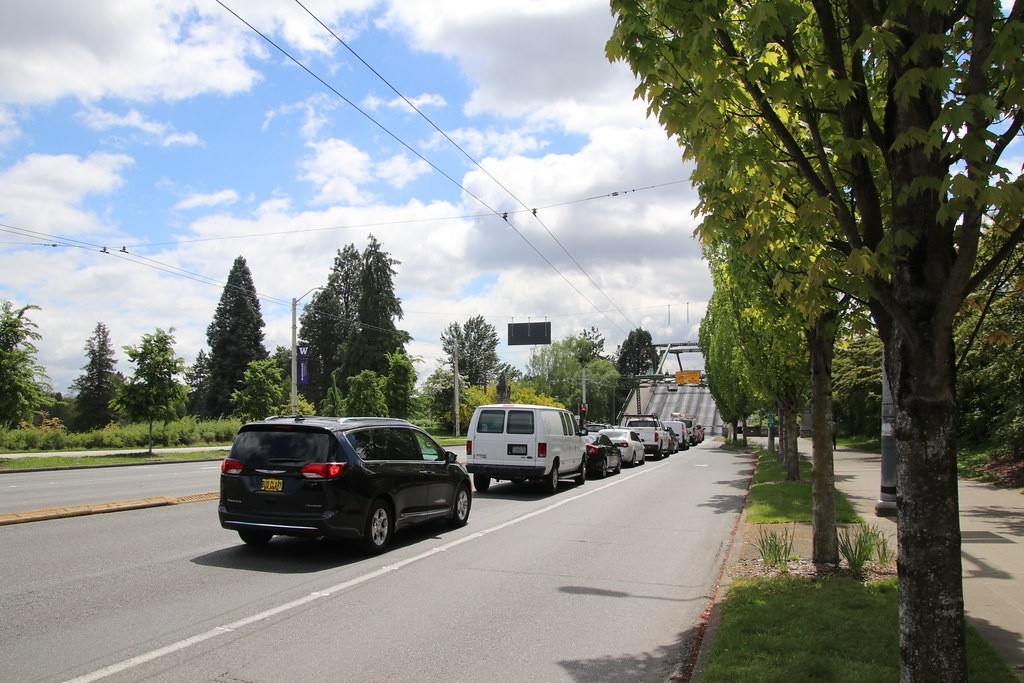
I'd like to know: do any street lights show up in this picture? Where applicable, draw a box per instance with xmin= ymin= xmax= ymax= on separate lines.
xmin=292 ymin=286 xmax=330 ymax=416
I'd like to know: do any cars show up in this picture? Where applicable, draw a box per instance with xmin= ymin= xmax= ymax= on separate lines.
xmin=598 ymin=428 xmax=646 ymax=467
xmin=578 ymin=432 xmax=623 ymax=479
xmin=664 ymin=426 xmax=679 ymax=454
xmin=666 ymin=384 xmax=677 ymax=391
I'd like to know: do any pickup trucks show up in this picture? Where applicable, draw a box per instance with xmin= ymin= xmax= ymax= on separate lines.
xmin=626 ymin=415 xmax=671 ymax=460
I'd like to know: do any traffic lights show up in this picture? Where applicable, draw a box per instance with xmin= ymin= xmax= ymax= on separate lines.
xmin=580 ymin=406 xmax=585 ymax=417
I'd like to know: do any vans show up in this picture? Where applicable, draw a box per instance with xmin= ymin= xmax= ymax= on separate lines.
xmin=660 ymin=421 xmax=690 ymax=451
xmin=674 ymin=418 xmax=700 ymax=445
xmin=467 ymin=404 xmax=588 ymax=494
xmin=218 ymin=415 xmax=473 ymax=554
